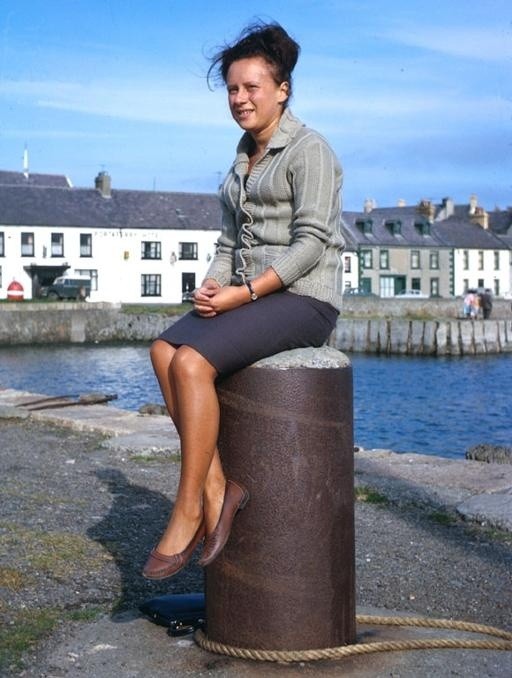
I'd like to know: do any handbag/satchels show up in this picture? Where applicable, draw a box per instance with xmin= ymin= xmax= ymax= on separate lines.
xmin=139 ymin=592 xmax=207 ymax=637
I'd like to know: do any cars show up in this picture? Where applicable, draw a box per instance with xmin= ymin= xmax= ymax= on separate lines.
xmin=394 ymin=286 xmax=431 ymax=299
xmin=455 ymin=287 xmax=493 ymax=300
xmin=343 ymin=287 xmax=381 ymax=299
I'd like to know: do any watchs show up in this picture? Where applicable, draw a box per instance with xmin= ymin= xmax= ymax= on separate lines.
xmin=245 ymin=280 xmax=258 ymax=301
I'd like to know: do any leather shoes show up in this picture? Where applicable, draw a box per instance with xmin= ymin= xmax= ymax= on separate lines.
xmin=142 ymin=518 xmax=205 ymax=580
xmin=198 ymin=478 xmax=250 ymax=568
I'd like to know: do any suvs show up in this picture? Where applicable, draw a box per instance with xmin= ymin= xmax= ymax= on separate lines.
xmin=37 ymin=276 xmax=93 ymax=301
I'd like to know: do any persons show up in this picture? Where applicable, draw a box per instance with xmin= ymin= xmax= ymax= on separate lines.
xmin=482 ymin=288 xmax=493 ymax=320
xmin=137 ymin=20 xmax=345 ymax=581
xmin=462 ymin=289 xmax=474 ymax=321
xmin=469 ymin=289 xmax=480 ymax=320
